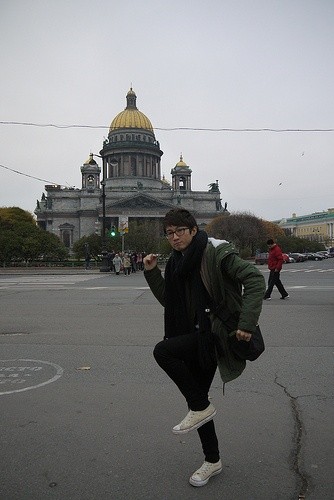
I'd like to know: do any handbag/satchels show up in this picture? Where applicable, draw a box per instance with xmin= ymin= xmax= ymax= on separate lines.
xmin=227 ymin=310 xmax=265 ymax=361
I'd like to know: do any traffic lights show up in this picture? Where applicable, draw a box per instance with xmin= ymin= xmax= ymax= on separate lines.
xmin=111 ymin=225 xmax=116 ymax=237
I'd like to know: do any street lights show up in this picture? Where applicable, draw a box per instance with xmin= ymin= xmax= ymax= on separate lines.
xmin=100 ymin=147 xmax=118 ymax=271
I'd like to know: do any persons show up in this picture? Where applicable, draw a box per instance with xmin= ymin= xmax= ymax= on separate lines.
xmin=261 ymin=238 xmax=290 ymax=301
xmin=144 ymin=209 xmax=267 ymax=488
xmin=112 ymin=250 xmax=147 ymax=277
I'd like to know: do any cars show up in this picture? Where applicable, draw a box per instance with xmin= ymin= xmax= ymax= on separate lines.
xmin=255 ymin=250 xmax=329 ymax=263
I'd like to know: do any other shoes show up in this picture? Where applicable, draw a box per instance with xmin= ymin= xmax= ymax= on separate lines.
xmin=280 ymin=295 xmax=290 ymax=300
xmin=263 ymin=297 xmax=271 ymax=301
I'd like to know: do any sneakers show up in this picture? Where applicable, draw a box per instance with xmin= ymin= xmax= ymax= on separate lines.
xmin=189 ymin=458 xmax=223 ymax=487
xmin=171 ymin=403 xmax=217 ymax=435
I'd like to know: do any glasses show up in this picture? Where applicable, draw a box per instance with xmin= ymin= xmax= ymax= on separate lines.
xmin=164 ymin=228 xmax=191 ymax=240
